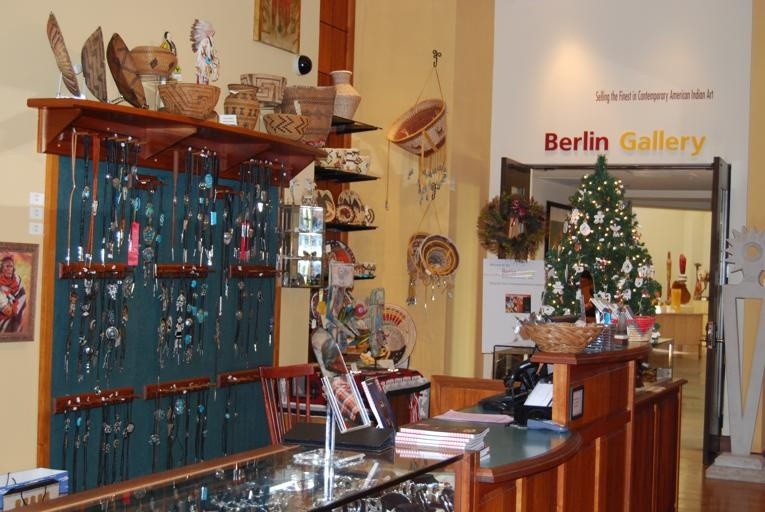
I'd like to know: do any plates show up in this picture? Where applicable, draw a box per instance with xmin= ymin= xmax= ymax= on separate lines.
xmin=317 ymin=189 xmax=366 ymax=226
xmin=81 ymin=26 xmax=109 ymax=103
xmin=105 ymin=32 xmax=146 ymax=108
xmin=46 ymin=10 xmax=81 ymax=97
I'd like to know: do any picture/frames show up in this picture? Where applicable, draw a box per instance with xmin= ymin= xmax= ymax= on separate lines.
xmin=570 ymin=385 xmax=584 ymax=421
xmin=0 ymin=242 xmax=40 ymax=342
xmin=543 ymin=199 xmax=573 ymax=258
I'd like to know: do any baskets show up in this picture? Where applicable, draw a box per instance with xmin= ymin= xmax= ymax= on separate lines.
xmin=612 ymin=316 xmax=655 ymax=342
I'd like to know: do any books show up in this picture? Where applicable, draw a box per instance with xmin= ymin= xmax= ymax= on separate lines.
xmin=589 ymin=295 xmax=634 ymax=321
xmin=359 ymin=376 xmax=397 ymax=431
xmin=433 ymin=408 xmax=515 ymax=424
xmin=284 ymin=419 xmax=491 ymax=461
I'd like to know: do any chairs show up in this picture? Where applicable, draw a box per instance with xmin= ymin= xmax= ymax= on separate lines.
xmin=259 ymin=362 xmax=321 ymax=446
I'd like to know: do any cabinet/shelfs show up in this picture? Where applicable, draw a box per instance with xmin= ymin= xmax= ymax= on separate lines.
xmin=3 ymin=442 xmax=480 ymax=512
xmin=315 ymin=112 xmax=384 ymax=279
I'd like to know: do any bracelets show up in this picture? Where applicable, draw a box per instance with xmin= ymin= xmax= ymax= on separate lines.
xmin=94 ymin=454 xmax=267 ymax=511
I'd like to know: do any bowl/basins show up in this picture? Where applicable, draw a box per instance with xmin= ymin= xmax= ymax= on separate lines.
xmin=263 ymin=113 xmax=311 ymax=141
xmin=130 ymin=44 xmax=177 ymax=77
xmin=318 ymin=147 xmax=370 ymax=176
xmin=157 ymin=83 xmax=222 ymax=121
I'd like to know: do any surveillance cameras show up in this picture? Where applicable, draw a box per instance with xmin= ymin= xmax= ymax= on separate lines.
xmin=293 ymin=55 xmax=312 ymax=76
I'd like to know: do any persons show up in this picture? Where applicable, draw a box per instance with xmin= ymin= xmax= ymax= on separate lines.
xmin=0 ymin=256 xmax=27 ymax=334
xmin=159 ymin=30 xmax=177 ymax=58
xmin=321 ymin=339 xmax=365 ymax=431
xmin=194 ymin=32 xmax=219 ymax=85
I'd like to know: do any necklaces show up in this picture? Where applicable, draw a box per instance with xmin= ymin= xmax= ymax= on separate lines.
xmin=57 ymin=130 xmax=284 ymax=296
xmin=64 ymin=269 xmax=269 ymax=385
xmin=62 ymin=385 xmax=238 ymax=494
